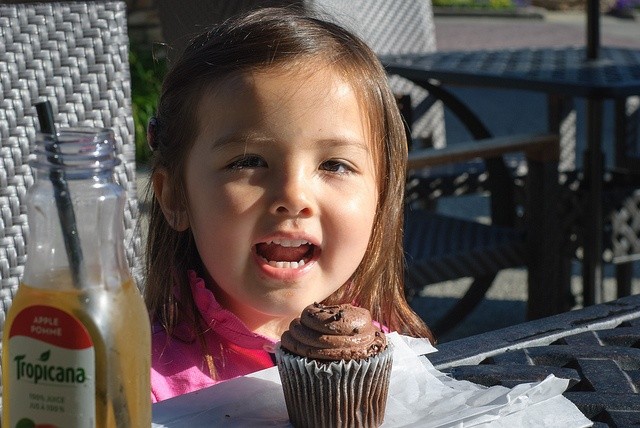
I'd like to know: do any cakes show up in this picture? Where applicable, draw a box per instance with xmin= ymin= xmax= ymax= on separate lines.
xmin=274 ymin=302 xmax=395 ymax=428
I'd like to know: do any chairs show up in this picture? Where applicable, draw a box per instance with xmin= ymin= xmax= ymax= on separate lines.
xmin=301 ymin=0 xmax=446 ymax=149
xmin=2 ymin=2 xmax=149 ymax=338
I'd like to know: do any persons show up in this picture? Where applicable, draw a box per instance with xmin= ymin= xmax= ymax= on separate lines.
xmin=136 ymin=7 xmax=438 ymax=408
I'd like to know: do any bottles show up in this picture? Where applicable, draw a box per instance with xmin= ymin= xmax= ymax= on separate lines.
xmin=3 ymin=126 xmax=152 ymax=427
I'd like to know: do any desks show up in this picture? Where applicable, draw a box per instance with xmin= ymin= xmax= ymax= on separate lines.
xmin=152 ymin=296 xmax=638 ymax=426
